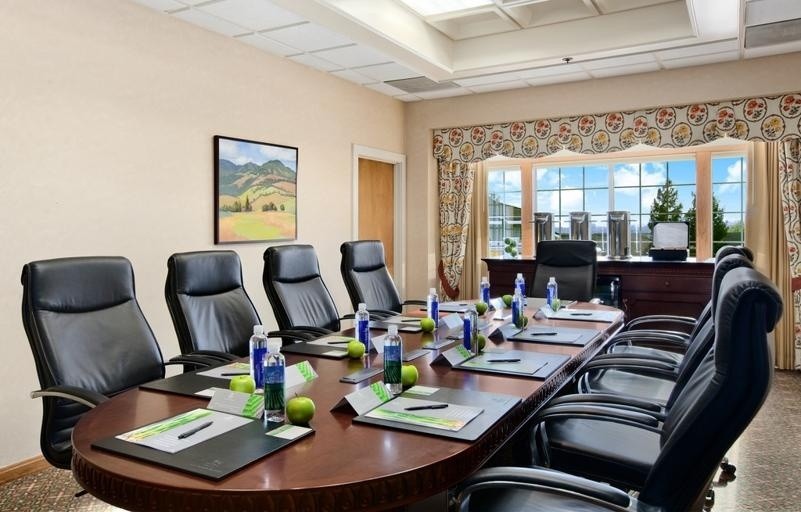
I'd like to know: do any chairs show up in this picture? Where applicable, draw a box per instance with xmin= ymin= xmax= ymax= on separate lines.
xmin=340 ymin=240 xmax=428 ymax=317
xmin=21 ymin=255 xmax=224 ymax=498
xmin=164 ymin=249 xmax=318 ymax=362
xmin=261 ymin=244 xmax=387 ymax=338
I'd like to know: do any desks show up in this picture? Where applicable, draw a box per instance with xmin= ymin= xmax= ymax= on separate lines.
xmin=69 ymin=296 xmax=625 ymax=511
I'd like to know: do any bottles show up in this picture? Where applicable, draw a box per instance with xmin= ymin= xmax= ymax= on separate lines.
xmin=481 ymin=277 xmax=491 ymax=308
xmin=384 ymin=325 xmax=404 ymax=394
xmin=546 ymin=276 xmax=559 ymax=307
xmin=355 ymin=302 xmax=371 ymax=355
xmin=262 ymin=341 xmax=288 ymax=423
xmin=464 ymin=304 xmax=479 ymax=356
xmin=427 ymin=288 xmax=440 ymax=328
xmin=512 ymin=288 xmax=524 ymax=325
xmin=515 ymin=272 xmax=527 ymax=305
xmin=249 ymin=324 xmax=268 ymax=388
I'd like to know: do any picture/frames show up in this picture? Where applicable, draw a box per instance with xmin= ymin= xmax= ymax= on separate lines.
xmin=214 ymin=134 xmax=298 ymax=244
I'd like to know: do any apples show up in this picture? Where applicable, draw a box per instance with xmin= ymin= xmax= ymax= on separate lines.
xmin=286 ymin=396 xmax=315 ymax=425
xmin=476 ymin=302 xmax=488 ymax=315
xmin=502 ymin=294 xmax=512 ymax=307
xmin=401 ymin=364 xmax=419 ymax=387
xmin=478 ymin=333 xmax=486 ymax=351
xmin=520 ymin=315 xmax=528 ymax=327
xmin=347 ymin=341 xmax=366 ymax=359
xmin=229 ymin=375 xmax=255 ymax=394
xmin=420 ymin=317 xmax=435 ymax=333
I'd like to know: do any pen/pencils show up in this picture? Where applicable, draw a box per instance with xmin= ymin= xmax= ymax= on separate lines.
xmin=571 ymin=314 xmax=592 ymax=315
xmin=487 ymin=360 xmax=521 ymax=362
xmin=404 ymin=404 xmax=448 ymax=410
xmin=531 ymin=333 xmax=557 ymax=335
xmin=460 ymin=304 xmax=476 ymax=306
xmin=178 ymin=421 xmax=213 ymax=439
xmin=328 ymin=342 xmax=349 ymax=344
xmin=401 ymin=320 xmax=421 ymax=322
xmin=222 ymin=373 xmax=249 ymax=376
